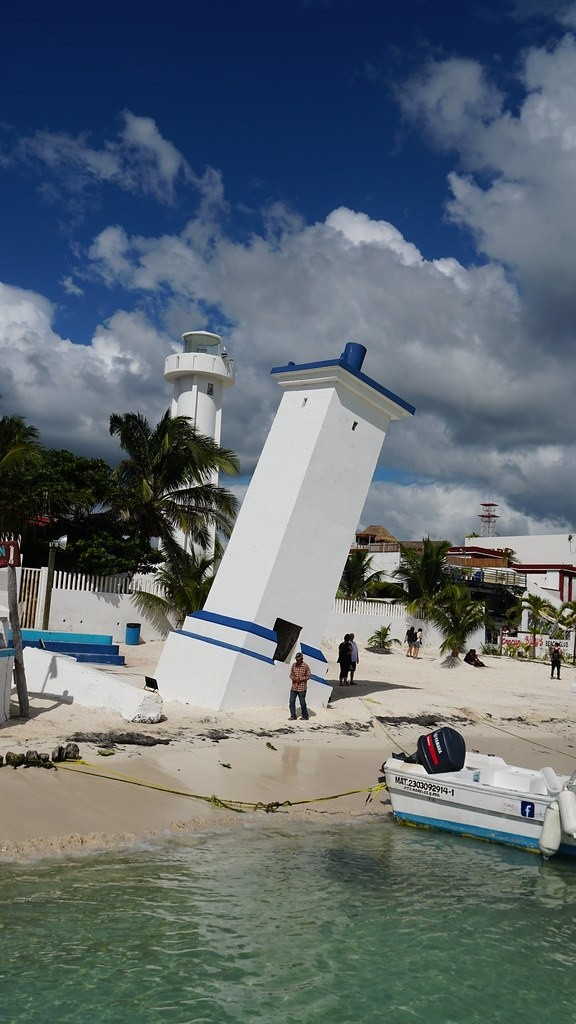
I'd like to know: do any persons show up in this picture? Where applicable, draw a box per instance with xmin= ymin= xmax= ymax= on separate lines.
xmin=349 ymin=633 xmax=359 ymax=686
xmin=464 ymin=649 xmax=486 ymax=666
xmin=502 ymin=627 xmax=509 ymax=636
xmin=338 ymin=634 xmax=353 ymax=686
xmin=404 ymin=627 xmax=414 ymax=657
xmin=288 ymin=653 xmax=310 ymax=721
xmin=550 ymin=642 xmax=562 ymax=679
xmin=413 ymin=628 xmax=422 ymax=658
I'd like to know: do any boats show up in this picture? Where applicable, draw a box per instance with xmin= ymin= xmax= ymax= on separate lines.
xmin=382 ymin=728 xmax=576 ymax=861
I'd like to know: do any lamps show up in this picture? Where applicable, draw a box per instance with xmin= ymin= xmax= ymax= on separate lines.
xmin=38 ymin=638 xmax=45 ymax=649
xmin=143 ymin=676 xmax=159 ymax=692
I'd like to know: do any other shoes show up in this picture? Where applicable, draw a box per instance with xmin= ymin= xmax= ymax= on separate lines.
xmin=300 ymin=716 xmax=309 ymax=720
xmin=345 ymin=682 xmax=349 ymax=686
xmin=350 ymin=682 xmax=357 ymax=685
xmin=288 ymin=716 xmax=297 ymax=720
xmin=556 ymin=678 xmax=561 ymax=680
xmin=339 ymin=683 xmax=346 ymax=686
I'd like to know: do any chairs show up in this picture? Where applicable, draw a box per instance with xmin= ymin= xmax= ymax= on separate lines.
xmin=540 ymin=767 xmax=563 ymax=793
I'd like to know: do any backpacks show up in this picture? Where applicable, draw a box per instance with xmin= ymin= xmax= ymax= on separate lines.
xmin=552 ymin=648 xmax=560 ymax=660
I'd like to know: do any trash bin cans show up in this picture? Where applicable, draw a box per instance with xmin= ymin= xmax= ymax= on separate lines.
xmin=125 ymin=623 xmax=141 ymax=645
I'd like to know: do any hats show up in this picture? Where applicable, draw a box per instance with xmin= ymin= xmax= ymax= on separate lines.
xmin=294 ymin=653 xmax=303 ymax=659
